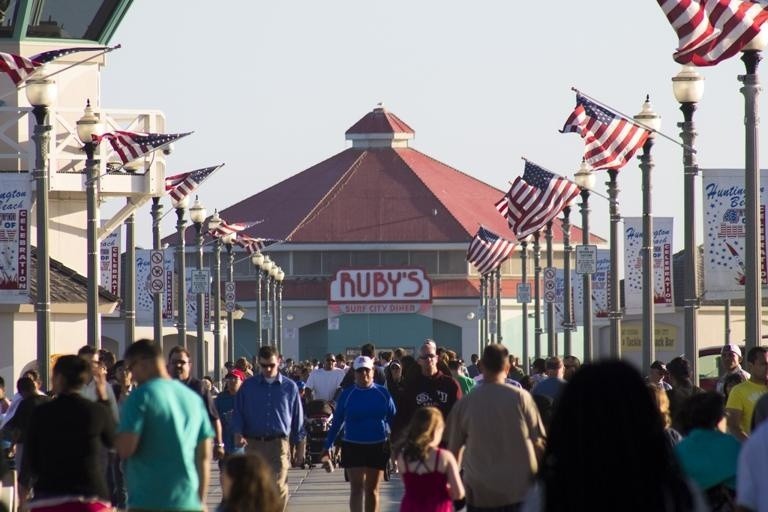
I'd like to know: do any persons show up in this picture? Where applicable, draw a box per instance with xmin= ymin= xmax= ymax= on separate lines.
xmin=0 ymin=341 xmax=768 ymax=511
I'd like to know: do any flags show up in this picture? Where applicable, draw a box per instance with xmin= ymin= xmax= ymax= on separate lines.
xmin=2 ymin=44 xmax=109 ymax=87
xmin=164 ymin=165 xmax=218 ymax=206
xmin=494 ymin=193 xmax=508 ymax=220
xmin=466 ymin=230 xmax=516 ymax=273
xmin=558 ymin=94 xmax=652 ymax=173
xmin=212 ymin=220 xmax=259 ymax=238
xmin=232 ymin=233 xmax=278 ymax=252
xmin=93 ymin=132 xmax=184 ymax=162
xmin=655 ymin=0 xmax=768 ymax=68
xmin=506 ymin=163 xmax=581 ymax=239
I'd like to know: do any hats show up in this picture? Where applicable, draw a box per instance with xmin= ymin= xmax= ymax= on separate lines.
xmin=295 ymin=381 xmax=306 ymax=389
xmin=353 ymin=356 xmax=374 ymax=370
xmin=225 ymin=368 xmax=245 ymax=381
xmin=721 ymin=344 xmax=742 ymax=358
xmin=650 ymin=361 xmax=691 ymax=375
xmin=390 ymin=360 xmax=401 ymax=369
xmin=532 ymin=356 xmax=580 ymax=374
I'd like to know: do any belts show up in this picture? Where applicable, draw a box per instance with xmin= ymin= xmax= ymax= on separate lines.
xmin=244 ymin=433 xmax=286 ymax=440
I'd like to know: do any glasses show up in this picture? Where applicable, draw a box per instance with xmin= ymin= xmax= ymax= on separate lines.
xmin=420 ymin=354 xmax=436 ymax=360
xmin=326 ymin=359 xmax=335 ymax=363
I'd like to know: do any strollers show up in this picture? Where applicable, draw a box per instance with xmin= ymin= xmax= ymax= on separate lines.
xmin=302 ymin=388 xmax=339 ymax=468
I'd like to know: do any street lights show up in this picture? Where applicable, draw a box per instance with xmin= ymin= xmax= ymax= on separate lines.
xmin=631 ymin=93 xmax=662 ymax=376
xmin=173 ymin=193 xmax=285 ymax=390
xmin=670 ymin=63 xmax=707 ymax=389
xmin=518 ymin=233 xmax=532 ymax=375
xmin=734 ymin=24 xmax=764 ymax=376
xmin=26 ymin=72 xmax=57 ymax=394
xmin=152 ymin=197 xmax=163 ymax=354
xmin=573 ymin=156 xmax=597 ymax=365
xmin=123 ymin=146 xmax=138 ymax=354
xmin=76 ymin=98 xmax=104 ymax=350
